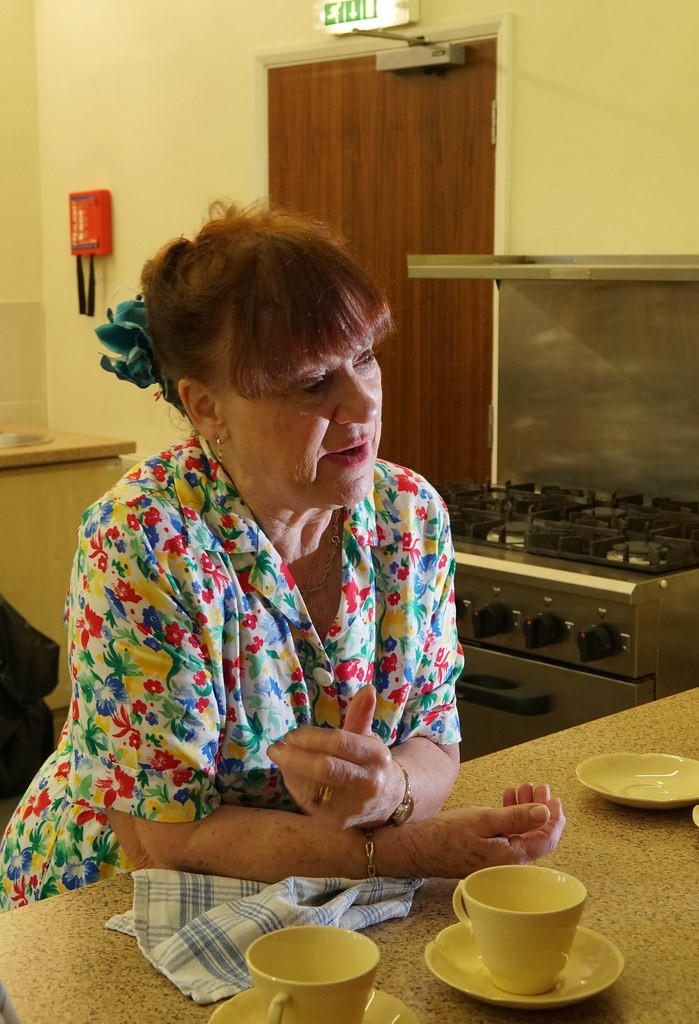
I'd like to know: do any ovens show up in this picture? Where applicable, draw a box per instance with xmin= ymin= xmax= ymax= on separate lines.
xmin=452 ymin=541 xmax=699 ymax=763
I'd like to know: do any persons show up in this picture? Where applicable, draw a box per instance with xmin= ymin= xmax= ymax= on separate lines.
xmin=0 ymin=214 xmax=567 ymax=934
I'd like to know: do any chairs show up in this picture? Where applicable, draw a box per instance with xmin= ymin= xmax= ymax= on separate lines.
xmin=0 ymin=575 xmax=65 ymax=813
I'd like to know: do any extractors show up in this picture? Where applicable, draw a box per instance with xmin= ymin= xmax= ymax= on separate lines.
xmin=407 ymin=253 xmax=699 ymax=282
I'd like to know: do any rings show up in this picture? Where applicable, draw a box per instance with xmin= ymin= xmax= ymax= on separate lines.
xmin=312 ymin=783 xmax=334 ymax=806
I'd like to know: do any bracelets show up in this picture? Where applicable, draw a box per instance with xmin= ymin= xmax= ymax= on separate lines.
xmin=363 ymin=826 xmax=376 ymax=879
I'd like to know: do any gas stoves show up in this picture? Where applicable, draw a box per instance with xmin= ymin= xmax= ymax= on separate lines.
xmin=431 ymin=477 xmax=699 ymax=577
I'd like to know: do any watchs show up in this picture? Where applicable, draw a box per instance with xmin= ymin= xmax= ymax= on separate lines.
xmin=385 ymin=753 xmax=414 ymax=827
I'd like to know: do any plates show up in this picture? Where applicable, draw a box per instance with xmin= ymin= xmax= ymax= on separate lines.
xmin=575 ymin=753 xmax=699 ymax=810
xmin=207 ymin=986 xmax=420 ymax=1024
xmin=424 ymin=923 xmax=626 ymax=1010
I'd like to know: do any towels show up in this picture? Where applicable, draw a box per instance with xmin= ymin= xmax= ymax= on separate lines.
xmin=99 ymin=867 xmax=425 ymax=1006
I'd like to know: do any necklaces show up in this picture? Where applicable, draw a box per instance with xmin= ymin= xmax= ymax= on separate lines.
xmin=297 ymin=507 xmax=343 ymax=600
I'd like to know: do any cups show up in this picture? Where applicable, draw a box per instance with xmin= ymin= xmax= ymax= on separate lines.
xmin=244 ymin=924 xmax=381 ymax=1024
xmin=452 ymin=865 xmax=587 ymax=996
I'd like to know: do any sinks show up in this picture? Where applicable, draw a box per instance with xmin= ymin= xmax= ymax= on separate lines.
xmin=0 ymin=432 xmax=54 ymax=448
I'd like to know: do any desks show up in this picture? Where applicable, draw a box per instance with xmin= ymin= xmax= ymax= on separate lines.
xmin=0 ymin=691 xmax=699 ymax=1024
